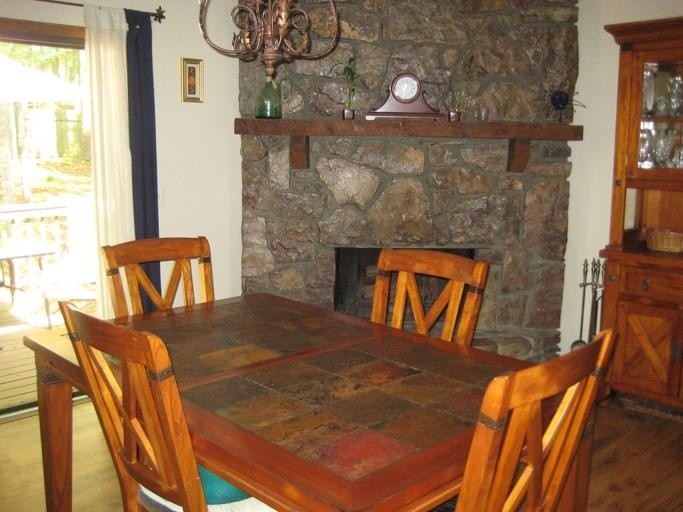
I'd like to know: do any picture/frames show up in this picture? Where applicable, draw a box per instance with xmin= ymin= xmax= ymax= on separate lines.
xmin=181 ymin=57 xmax=204 ymax=103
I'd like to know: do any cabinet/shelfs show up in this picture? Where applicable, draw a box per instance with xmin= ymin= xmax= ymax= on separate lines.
xmin=599 ymin=15 xmax=683 ymax=409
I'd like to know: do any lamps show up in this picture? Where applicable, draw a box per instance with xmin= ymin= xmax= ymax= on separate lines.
xmin=199 ymin=0 xmax=339 ymax=76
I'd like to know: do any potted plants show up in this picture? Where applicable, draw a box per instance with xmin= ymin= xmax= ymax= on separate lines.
xmin=449 ymin=54 xmax=474 ymax=121
xmin=342 ymin=56 xmax=360 ymax=120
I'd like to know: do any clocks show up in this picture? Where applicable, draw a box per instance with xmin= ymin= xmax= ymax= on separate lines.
xmin=366 ymin=73 xmax=447 ymax=122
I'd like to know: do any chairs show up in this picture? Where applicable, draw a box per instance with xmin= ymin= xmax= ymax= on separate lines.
xmin=431 ymin=328 xmax=617 ymax=512
xmin=100 ymin=236 xmax=215 ymax=318
xmin=60 ymin=301 xmax=275 ymax=512
xmin=370 ymin=248 xmax=490 ymax=345
